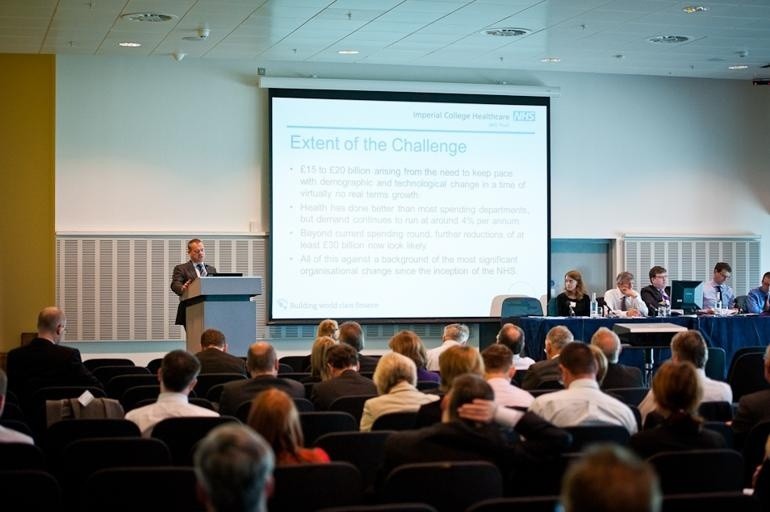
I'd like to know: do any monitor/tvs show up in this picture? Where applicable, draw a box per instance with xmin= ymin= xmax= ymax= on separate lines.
xmin=670 ymin=280 xmax=704 ymax=315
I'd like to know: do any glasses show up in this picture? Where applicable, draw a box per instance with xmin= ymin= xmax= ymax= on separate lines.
xmin=719 ymin=272 xmax=731 ymax=278
xmin=655 ymin=275 xmax=668 ymax=278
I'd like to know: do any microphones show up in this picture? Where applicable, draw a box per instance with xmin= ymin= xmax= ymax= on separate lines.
xmin=565 ymin=300 xmax=578 ymax=308
xmin=646 ymin=301 xmax=658 ymax=312
xmin=598 ymin=301 xmax=613 ymax=319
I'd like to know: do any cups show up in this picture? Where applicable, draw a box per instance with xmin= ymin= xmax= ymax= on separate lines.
xmin=658 ymin=306 xmax=666 ymax=317
xmin=598 ymin=307 xmax=603 ymax=317
xmin=721 ymin=306 xmax=728 ymax=317
xmin=664 ymin=300 xmax=672 ymax=316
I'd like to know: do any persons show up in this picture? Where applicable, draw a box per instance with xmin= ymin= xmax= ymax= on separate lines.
xmin=641 ymin=266 xmax=671 ymax=315
xmin=744 ymin=272 xmax=770 ymax=314
xmin=603 ymin=272 xmax=649 ymax=319
xmin=170 ymin=239 xmax=216 ymax=333
xmin=2 ymin=306 xmax=770 ymax=511
xmin=693 ymin=262 xmax=743 ymax=315
xmin=557 ymin=270 xmax=590 ymax=315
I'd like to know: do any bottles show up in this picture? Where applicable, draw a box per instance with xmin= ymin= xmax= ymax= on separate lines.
xmin=713 ymin=292 xmax=722 ymax=317
xmin=590 ymin=292 xmax=598 ymax=318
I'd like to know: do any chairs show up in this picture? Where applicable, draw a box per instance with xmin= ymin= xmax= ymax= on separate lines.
xmin=548 ymin=297 xmax=557 ymax=316
xmin=735 ymin=296 xmax=747 ymax=314
xmin=501 ymin=297 xmax=543 ymax=319
xmin=706 ymin=348 xmax=726 ymax=380
xmin=726 ymin=345 xmax=768 ymax=402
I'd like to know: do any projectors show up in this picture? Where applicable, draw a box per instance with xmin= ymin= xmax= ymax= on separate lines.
xmin=612 ymin=322 xmax=689 ymax=348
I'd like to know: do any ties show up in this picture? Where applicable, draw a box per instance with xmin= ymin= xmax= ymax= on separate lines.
xmin=195 ymin=264 xmax=207 ymax=277
xmin=658 ymin=288 xmax=668 ymax=300
xmin=620 ymin=296 xmax=627 ymax=311
xmin=715 ymin=286 xmax=723 ymax=309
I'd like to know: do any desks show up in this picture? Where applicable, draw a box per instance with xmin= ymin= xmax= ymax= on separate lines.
xmin=521 ymin=313 xmax=770 ymax=377
